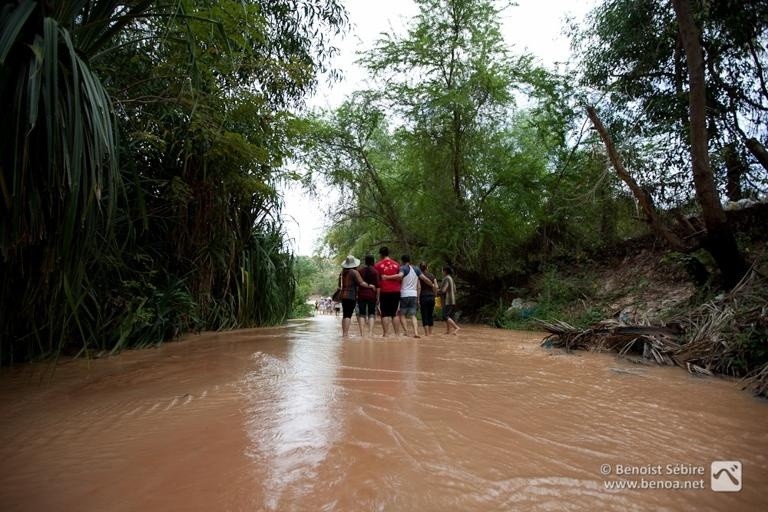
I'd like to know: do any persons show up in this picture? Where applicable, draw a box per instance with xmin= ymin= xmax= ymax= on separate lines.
xmin=436 ymin=266 xmax=462 ymax=335
xmin=417 ymin=262 xmax=438 ymax=337
xmin=314 ymin=289 xmax=376 ymax=324
xmin=338 ymin=255 xmax=376 ymax=339
xmin=354 ymin=255 xmax=380 ymax=338
xmin=373 ymin=246 xmax=401 ymax=337
xmin=381 ymin=255 xmax=433 ymax=337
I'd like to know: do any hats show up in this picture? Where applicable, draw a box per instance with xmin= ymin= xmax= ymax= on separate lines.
xmin=341 ymin=255 xmax=360 ymax=269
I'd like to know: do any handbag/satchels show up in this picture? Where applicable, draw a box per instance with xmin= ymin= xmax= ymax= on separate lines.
xmin=332 ymin=288 xmax=356 ymax=301
